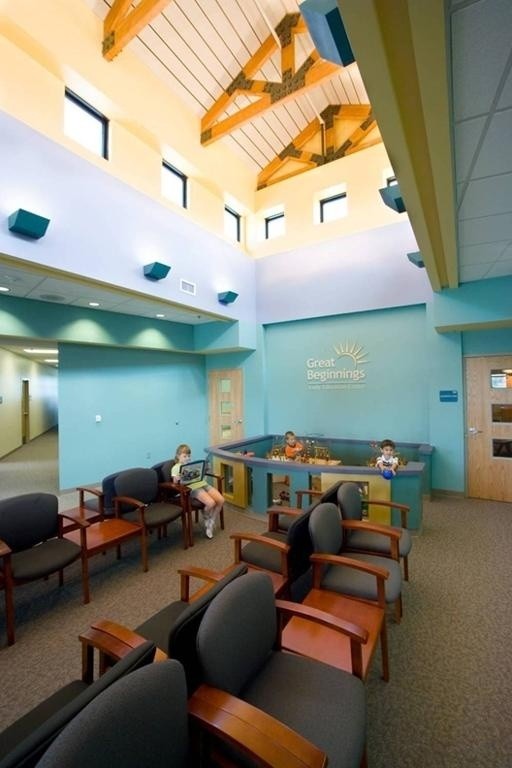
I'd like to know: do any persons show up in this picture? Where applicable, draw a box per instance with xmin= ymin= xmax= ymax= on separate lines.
xmin=375 ymin=440 xmax=399 ymax=476
xmin=171 ymin=444 xmax=225 ymax=539
xmin=284 ymin=431 xmax=304 ymax=459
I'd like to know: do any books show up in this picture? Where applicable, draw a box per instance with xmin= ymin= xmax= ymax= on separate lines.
xmin=178 ymin=450 xmax=212 ymax=486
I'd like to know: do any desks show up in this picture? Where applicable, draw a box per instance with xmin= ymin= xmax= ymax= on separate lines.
xmin=269 ymin=454 xmax=341 ymax=466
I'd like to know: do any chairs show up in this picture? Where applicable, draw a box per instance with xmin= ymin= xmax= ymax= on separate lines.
xmin=195 ymin=572 xmax=371 ymax=767
xmin=1 ymin=461 xmax=227 ymax=646
xmin=31 ymin=658 xmax=310 ymax=766
xmin=189 ymin=474 xmax=410 ymax=685
xmin=1 ymin=626 xmax=159 ymax=767
xmin=89 ymin=559 xmax=250 ymax=677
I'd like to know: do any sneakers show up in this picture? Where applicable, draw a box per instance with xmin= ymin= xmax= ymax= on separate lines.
xmin=203 ymin=510 xmax=216 ymax=538
